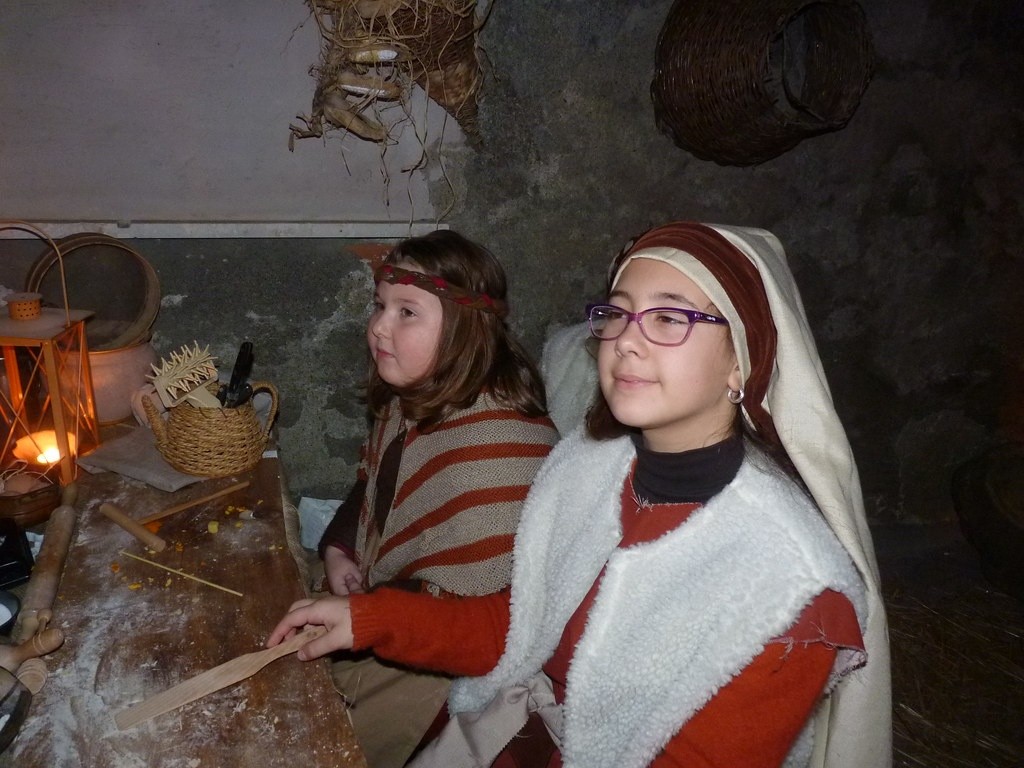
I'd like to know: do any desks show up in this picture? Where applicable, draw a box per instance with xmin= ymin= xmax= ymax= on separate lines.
xmin=0 ymin=417 xmax=366 ymax=768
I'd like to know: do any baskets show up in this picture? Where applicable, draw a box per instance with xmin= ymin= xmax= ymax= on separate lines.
xmin=140 ymin=379 xmax=279 ymax=479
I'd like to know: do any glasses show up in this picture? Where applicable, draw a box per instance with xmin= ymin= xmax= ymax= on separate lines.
xmin=585 ymin=302 xmax=729 ymax=347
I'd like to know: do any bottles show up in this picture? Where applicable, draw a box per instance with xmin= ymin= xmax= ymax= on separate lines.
xmin=40 ymin=318 xmax=156 ymax=427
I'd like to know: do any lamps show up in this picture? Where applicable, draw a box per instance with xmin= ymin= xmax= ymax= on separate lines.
xmin=0 ymin=221 xmax=100 ymax=485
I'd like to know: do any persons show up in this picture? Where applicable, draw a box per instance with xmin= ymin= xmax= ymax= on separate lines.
xmin=268 ymin=221 xmax=892 ymax=768
xmin=311 ymin=229 xmax=557 ymax=768
